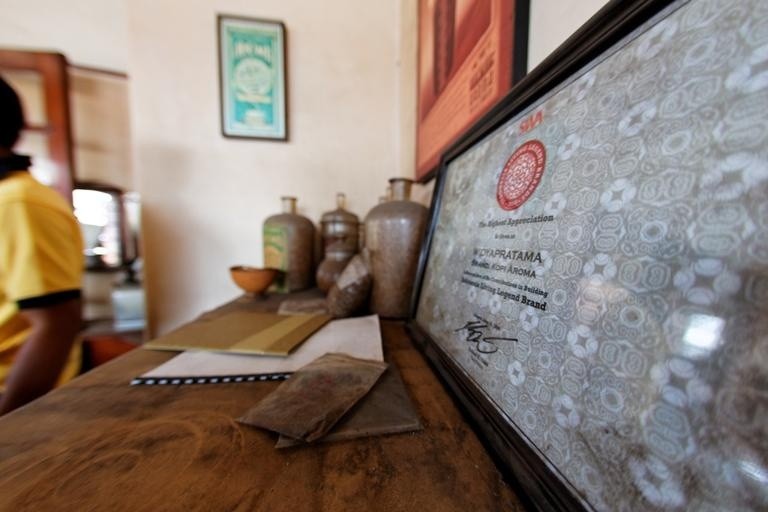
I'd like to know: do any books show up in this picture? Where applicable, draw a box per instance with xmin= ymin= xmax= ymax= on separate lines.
xmin=144 ymin=308 xmax=337 ymax=358
xmin=130 ymin=312 xmax=384 ymax=386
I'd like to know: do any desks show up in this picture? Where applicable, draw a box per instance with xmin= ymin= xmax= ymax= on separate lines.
xmin=1 ymin=287 xmax=526 ymax=511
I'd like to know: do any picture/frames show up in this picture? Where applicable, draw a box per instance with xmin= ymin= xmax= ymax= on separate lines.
xmin=217 ymin=13 xmax=289 ymax=143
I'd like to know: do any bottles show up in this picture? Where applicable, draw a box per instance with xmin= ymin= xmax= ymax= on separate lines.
xmin=263 ymin=197 xmax=316 ymax=294
xmin=363 ymin=178 xmax=430 ymax=319
xmin=316 ymin=192 xmax=359 ymax=296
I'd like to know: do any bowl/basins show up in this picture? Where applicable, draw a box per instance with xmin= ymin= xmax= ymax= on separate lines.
xmin=228 ymin=266 xmax=279 ymax=299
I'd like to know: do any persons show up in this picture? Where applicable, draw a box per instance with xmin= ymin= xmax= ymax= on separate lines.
xmin=0 ymin=74 xmax=88 ymax=413
